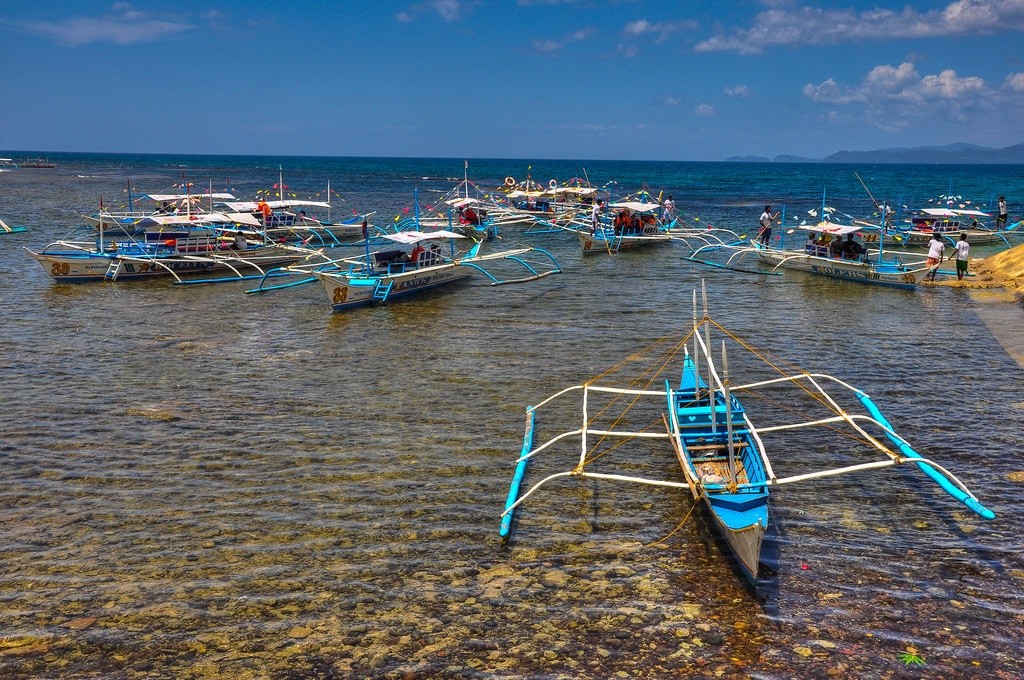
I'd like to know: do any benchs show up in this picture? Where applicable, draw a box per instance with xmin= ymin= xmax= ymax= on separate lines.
xmin=144 ymin=231 xmax=220 ymax=255
xmin=643 ymin=223 xmax=657 ymax=234
xmin=804 ymin=244 xmax=831 ymax=257
xmin=912 ymin=218 xmax=960 ymax=233
xmin=675 ymin=393 xmax=746 ymax=450
xmin=263 ymin=215 xmax=295 ymax=228
xmin=374 ymin=246 xmax=442 ymax=273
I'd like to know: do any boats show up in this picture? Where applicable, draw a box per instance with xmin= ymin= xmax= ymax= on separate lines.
xmin=22 ymin=212 xmax=342 ymax=286
xmin=498 ymin=275 xmax=997 ymax=588
xmin=535 ymin=175 xmax=613 ymax=214
xmin=144 ymin=175 xmax=236 ymax=215
xmin=524 ymin=201 xmax=746 ymax=255
xmin=0 ymin=220 xmax=27 ymax=234
xmin=244 ymin=230 xmax=563 ymax=310
xmin=390 ymin=181 xmax=553 ymax=241
xmin=680 ymin=221 xmax=978 ymax=291
xmin=504 ymin=174 xmax=546 ymax=210
xmin=845 ymin=207 xmax=1024 ymax=248
xmin=207 ymin=200 xmax=394 ymax=248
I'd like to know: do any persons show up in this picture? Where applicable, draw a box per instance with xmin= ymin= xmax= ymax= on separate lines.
xmin=590 ymin=198 xmax=603 ymax=236
xmin=878 ymin=201 xmax=891 ymax=234
xmin=233 ymin=231 xmax=248 ymax=250
xmin=662 ymin=195 xmax=675 ymax=228
xmin=994 ymin=197 xmax=1008 ymax=233
xmin=804 ymin=232 xmax=862 ymax=261
xmin=948 ymin=234 xmax=969 ymax=280
xmin=925 ymin=233 xmax=945 ymax=282
xmin=295 ymin=211 xmax=305 ymax=221
xmin=614 ymin=212 xmax=655 ymax=236
xmin=760 ymin=206 xmax=778 ymax=249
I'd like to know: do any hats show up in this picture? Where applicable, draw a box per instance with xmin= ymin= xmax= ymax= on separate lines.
xmin=808 ymin=232 xmax=817 ymax=236
